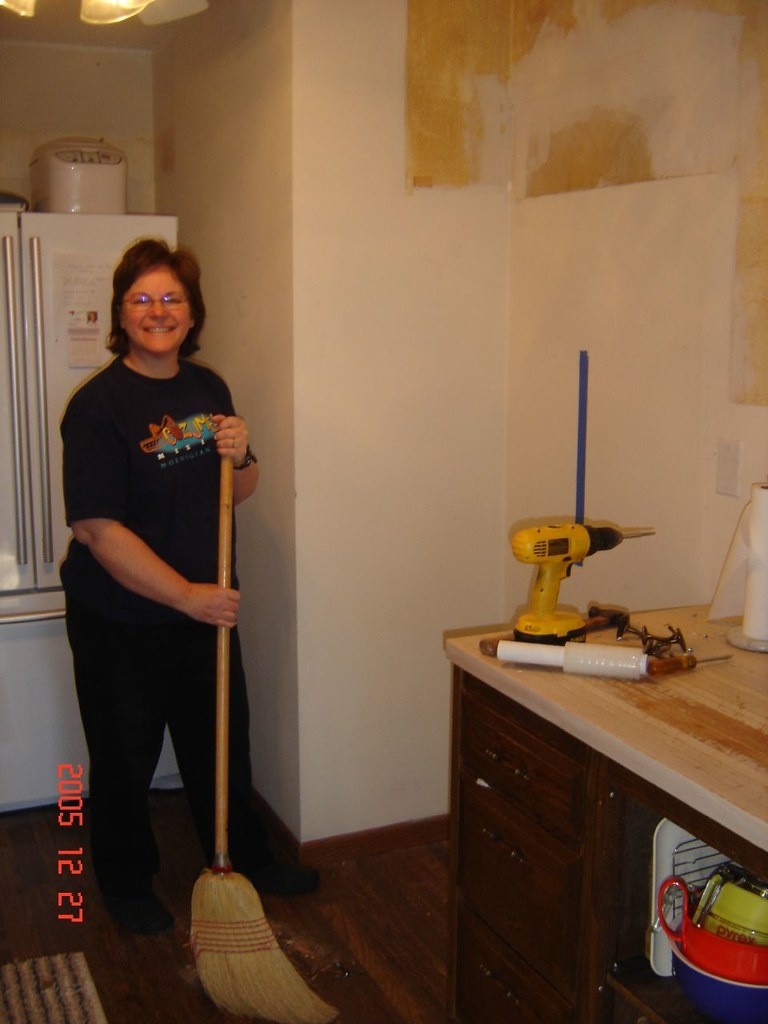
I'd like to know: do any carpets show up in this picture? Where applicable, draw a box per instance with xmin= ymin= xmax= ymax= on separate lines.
xmin=1 ymin=956 xmax=110 ymax=1024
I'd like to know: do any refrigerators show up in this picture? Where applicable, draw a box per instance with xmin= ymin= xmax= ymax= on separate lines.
xmin=0 ymin=210 xmax=179 ymax=817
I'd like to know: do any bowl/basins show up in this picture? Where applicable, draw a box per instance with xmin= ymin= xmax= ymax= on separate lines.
xmin=667 ymin=917 xmax=768 ymax=1024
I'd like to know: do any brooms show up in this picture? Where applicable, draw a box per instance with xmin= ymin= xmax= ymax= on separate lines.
xmin=190 ymin=420 xmax=339 ymax=1023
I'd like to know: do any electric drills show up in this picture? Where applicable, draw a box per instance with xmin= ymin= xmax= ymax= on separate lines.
xmin=510 ymin=522 xmax=656 ymax=647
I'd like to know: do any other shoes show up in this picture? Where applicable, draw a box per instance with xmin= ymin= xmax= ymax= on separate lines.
xmin=234 ymin=863 xmax=320 ymax=894
xmin=104 ymin=891 xmax=176 ymax=935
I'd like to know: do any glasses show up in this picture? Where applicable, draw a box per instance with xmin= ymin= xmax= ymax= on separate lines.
xmin=120 ymin=291 xmax=193 ymax=309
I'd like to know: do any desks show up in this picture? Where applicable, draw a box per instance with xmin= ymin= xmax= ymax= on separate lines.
xmin=442 ymin=603 xmax=768 ymax=1024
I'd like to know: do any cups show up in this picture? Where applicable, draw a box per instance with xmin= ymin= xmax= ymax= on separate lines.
xmin=657 ymin=874 xmax=768 ymax=986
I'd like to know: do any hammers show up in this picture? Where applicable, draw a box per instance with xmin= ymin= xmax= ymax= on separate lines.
xmin=479 ymin=606 xmax=631 ymax=657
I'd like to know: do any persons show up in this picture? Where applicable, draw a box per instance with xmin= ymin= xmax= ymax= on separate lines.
xmin=87 ymin=312 xmax=98 ymax=324
xmin=59 ymin=238 xmax=319 ymax=933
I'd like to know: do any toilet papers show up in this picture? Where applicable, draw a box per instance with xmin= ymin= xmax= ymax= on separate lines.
xmin=707 ymin=482 xmax=768 ymax=641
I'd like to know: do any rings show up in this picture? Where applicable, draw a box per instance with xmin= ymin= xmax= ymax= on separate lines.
xmin=232 ymin=438 xmax=239 ymax=448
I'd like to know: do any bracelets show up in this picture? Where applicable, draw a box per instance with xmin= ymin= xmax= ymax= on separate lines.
xmin=234 ymin=445 xmax=258 ymax=470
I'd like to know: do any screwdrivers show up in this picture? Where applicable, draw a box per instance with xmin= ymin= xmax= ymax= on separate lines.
xmin=647 ymin=655 xmax=733 ymax=678
